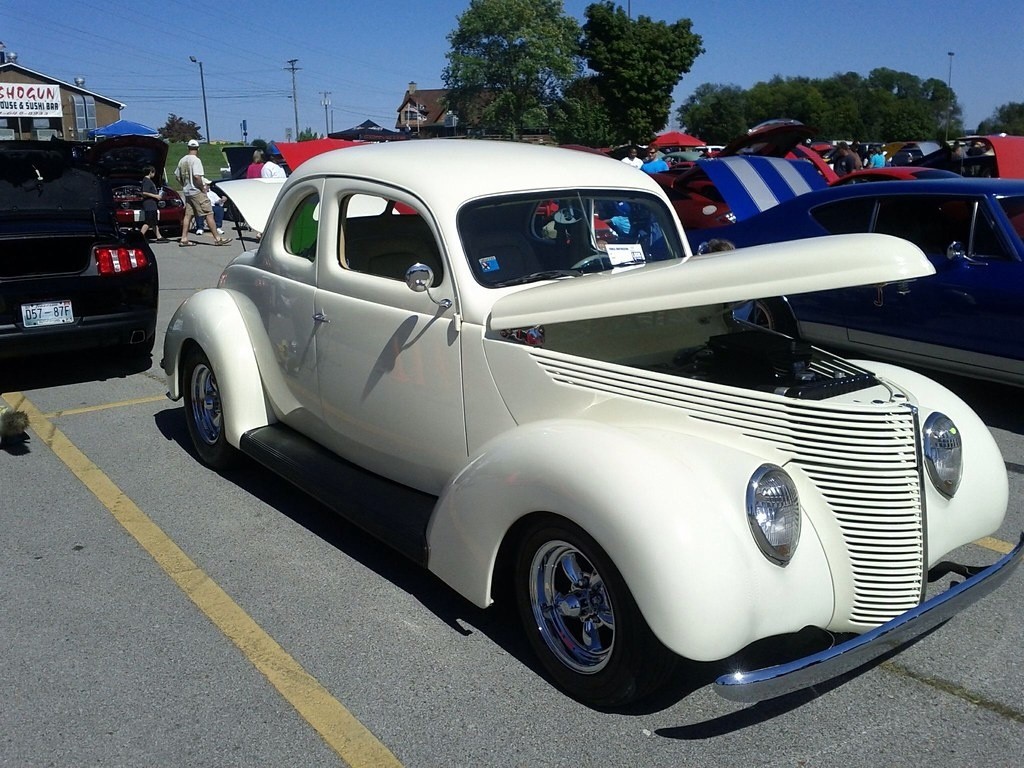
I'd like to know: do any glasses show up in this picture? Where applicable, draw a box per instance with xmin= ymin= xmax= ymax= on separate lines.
xmin=648 ymin=152 xmax=656 ymax=154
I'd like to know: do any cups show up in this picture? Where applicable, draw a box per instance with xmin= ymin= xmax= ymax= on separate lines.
xmin=219 ymin=200 xmax=224 ymax=207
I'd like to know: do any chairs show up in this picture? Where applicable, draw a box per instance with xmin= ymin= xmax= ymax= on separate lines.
xmin=467 ymin=231 xmax=543 ymax=286
xmin=355 ymin=237 xmax=444 ymax=291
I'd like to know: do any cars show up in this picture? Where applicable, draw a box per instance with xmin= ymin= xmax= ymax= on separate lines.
xmin=163 ymin=140 xmax=1024 ymax=707
xmin=210 ymin=140 xmax=419 ymax=222
xmin=646 ymin=156 xmax=1023 ymax=388
xmin=0 ymin=135 xmax=185 ymax=370
xmin=558 ymin=118 xmax=1024 ymax=239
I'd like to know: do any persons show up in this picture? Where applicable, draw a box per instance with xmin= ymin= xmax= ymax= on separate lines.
xmin=173 ymin=139 xmax=233 ymax=247
xmin=825 ymin=142 xmax=886 ymax=178
xmin=195 ymin=176 xmax=225 ymax=234
xmin=641 ymin=144 xmax=670 ymax=174
xmin=140 ymin=165 xmax=170 ymax=243
xmin=596 ymin=198 xmax=631 ymax=238
xmin=620 ymin=148 xmax=644 ymax=171
xmin=702 ymin=237 xmax=754 ymax=320
xmin=247 ymin=149 xmax=265 ymax=179
xmin=965 ymin=140 xmax=987 ymax=156
xmin=952 ymin=141 xmax=967 ymax=158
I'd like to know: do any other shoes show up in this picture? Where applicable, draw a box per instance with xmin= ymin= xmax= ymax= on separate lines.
xmin=156 ymin=237 xmax=169 ymax=242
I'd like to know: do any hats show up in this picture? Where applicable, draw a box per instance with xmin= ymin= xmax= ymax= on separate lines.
xmin=839 ymin=142 xmax=850 ymax=149
xmin=270 ymin=144 xmax=281 ymax=155
xmin=617 ymin=201 xmax=632 ymax=218
xmin=188 ymin=139 xmax=199 ymax=148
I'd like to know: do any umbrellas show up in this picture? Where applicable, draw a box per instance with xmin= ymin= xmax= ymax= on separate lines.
xmin=651 ymin=131 xmax=707 ymax=162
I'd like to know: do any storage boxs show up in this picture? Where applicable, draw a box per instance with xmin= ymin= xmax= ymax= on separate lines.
xmin=30 ymin=118 xmax=56 ymax=141
xmin=0 ymin=118 xmax=15 ymax=141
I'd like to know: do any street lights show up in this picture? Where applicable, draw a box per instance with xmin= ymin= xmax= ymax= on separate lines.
xmin=944 ymin=51 xmax=954 ymax=144
xmin=189 ymin=56 xmax=210 ymax=144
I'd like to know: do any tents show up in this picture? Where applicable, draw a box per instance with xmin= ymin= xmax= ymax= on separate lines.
xmin=86 ymin=118 xmax=168 ymax=184
xmin=328 ymin=119 xmax=410 ymax=141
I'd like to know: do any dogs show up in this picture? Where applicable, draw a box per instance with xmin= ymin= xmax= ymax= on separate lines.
xmin=0 ymin=406 xmax=30 ymax=444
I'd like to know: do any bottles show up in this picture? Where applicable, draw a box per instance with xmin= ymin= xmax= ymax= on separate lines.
xmin=159 ymin=187 xmax=165 ymax=198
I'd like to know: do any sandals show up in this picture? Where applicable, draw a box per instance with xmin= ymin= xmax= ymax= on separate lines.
xmin=180 ymin=240 xmax=196 ymax=246
xmin=215 ymin=237 xmax=233 ymax=247
